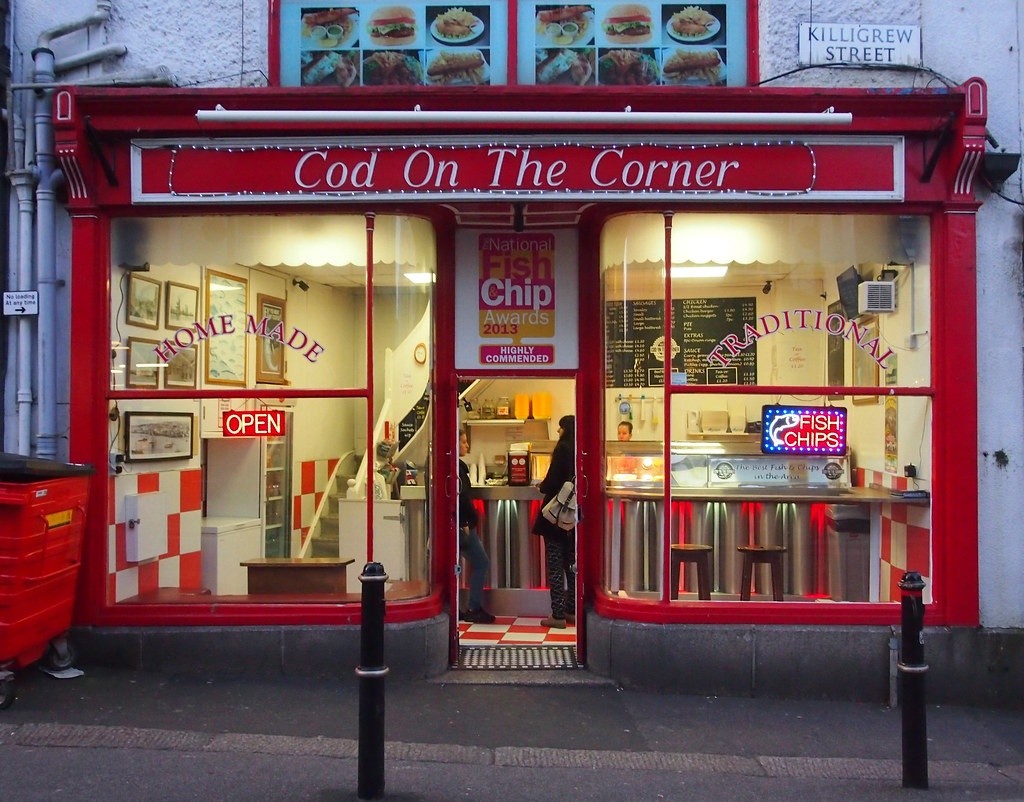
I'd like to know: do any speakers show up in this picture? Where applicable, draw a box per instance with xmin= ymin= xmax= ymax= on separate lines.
xmin=116 ymin=222 xmax=150 ymax=272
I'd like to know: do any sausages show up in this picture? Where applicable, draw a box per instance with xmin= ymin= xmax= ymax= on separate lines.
xmin=663 ymin=58 xmax=721 ymax=72
xmin=427 ymin=59 xmax=484 ymax=75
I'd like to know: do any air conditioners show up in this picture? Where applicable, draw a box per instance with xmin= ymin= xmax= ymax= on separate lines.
xmin=856 ymin=281 xmax=893 ymax=315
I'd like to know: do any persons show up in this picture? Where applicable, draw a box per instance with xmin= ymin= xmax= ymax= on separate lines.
xmin=531 ymin=415 xmax=576 ymax=629
xmin=607 ymin=421 xmax=641 ymax=475
xmin=459 ymin=429 xmax=497 ymax=624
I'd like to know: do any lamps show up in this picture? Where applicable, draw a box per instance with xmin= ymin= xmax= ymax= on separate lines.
xmin=976 ymin=152 xmax=1022 ymax=193
xmin=292 ymin=278 xmax=310 ymax=292
xmin=762 ymin=280 xmax=773 ymax=295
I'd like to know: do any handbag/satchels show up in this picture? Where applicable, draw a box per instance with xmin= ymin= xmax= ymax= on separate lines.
xmin=541 ymin=482 xmax=577 ymax=531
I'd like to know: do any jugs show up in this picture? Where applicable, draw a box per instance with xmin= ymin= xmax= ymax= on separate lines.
xmin=494 ymin=455 xmax=508 ymax=477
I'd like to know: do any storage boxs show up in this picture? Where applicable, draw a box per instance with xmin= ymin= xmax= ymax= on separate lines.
xmin=514 ymin=393 xmax=531 ymax=418
xmin=530 ymin=392 xmax=553 ymax=419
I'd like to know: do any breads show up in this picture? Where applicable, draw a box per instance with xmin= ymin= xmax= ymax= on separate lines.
xmin=436 ymin=23 xmax=470 ymax=36
xmin=671 ymin=22 xmax=706 ymax=34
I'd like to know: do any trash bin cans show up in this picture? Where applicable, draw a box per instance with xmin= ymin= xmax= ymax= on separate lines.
xmin=0 ymin=452 xmax=97 ymax=710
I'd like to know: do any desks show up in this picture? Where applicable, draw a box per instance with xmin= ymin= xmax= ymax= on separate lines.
xmin=236 ymin=555 xmax=356 ymax=595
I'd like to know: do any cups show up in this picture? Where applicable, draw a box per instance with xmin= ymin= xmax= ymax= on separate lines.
xmin=686 ymin=409 xmax=700 ymax=433
xmin=469 ymin=454 xmax=487 ymax=485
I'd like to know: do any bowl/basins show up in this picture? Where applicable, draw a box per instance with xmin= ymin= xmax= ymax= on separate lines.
xmin=484 ymin=478 xmax=509 ymax=486
xmin=613 ymin=474 xmax=637 ymax=480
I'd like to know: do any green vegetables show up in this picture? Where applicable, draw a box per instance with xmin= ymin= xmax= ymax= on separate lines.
xmin=362 ymin=60 xmax=380 ymax=84
xmin=597 ymin=54 xmax=654 ymax=77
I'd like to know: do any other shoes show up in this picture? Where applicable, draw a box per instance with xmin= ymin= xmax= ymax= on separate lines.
xmin=540 ymin=616 xmax=566 ymax=629
xmin=464 ymin=607 xmax=496 ymax=624
xmin=459 ymin=611 xmax=466 ymax=620
xmin=562 ymin=611 xmax=575 ymax=624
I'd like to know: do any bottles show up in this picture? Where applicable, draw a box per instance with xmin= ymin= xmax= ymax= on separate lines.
xmin=496 ymin=397 xmax=512 ymax=419
xmin=468 ymin=398 xmax=481 ymax=419
xmin=482 ymin=398 xmax=497 ymax=419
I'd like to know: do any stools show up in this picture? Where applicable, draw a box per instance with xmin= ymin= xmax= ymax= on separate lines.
xmin=736 ymin=542 xmax=789 ymax=602
xmin=671 ymin=542 xmax=715 ymax=600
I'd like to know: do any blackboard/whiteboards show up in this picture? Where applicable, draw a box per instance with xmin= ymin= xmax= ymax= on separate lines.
xmin=606 ymin=297 xmax=757 ymax=388
xmin=828 ymin=300 xmax=844 ymax=401
xmin=852 ymin=315 xmax=879 ymax=405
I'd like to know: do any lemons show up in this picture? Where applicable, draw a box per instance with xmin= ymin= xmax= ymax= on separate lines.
xmin=320 ymin=40 xmax=338 ymax=47
xmin=551 ymin=37 xmax=573 ymax=44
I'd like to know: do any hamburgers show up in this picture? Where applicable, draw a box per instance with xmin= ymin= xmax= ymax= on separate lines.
xmin=602 ymin=4 xmax=653 ymax=42
xmin=367 ymin=6 xmax=416 ymax=45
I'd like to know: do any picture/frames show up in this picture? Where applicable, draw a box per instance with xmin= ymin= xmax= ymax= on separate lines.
xmin=204 ymin=267 xmax=249 ymax=386
xmin=125 ymin=335 xmax=161 ymax=390
xmin=163 ymin=280 xmax=201 ymax=333
xmin=255 ymin=292 xmax=287 ymax=385
xmin=125 ymin=271 xmax=162 ymax=329
xmin=124 ymin=410 xmax=194 ymax=463
xmin=163 ymin=339 xmax=199 ymax=388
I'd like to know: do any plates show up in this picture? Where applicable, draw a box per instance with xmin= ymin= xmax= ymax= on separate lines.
xmin=534 ymin=4 xmax=726 ymax=84
xmin=300 ymin=10 xmax=490 ymax=86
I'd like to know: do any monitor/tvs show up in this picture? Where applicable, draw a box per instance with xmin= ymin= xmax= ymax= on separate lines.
xmin=836 ymin=264 xmax=864 ymax=321
